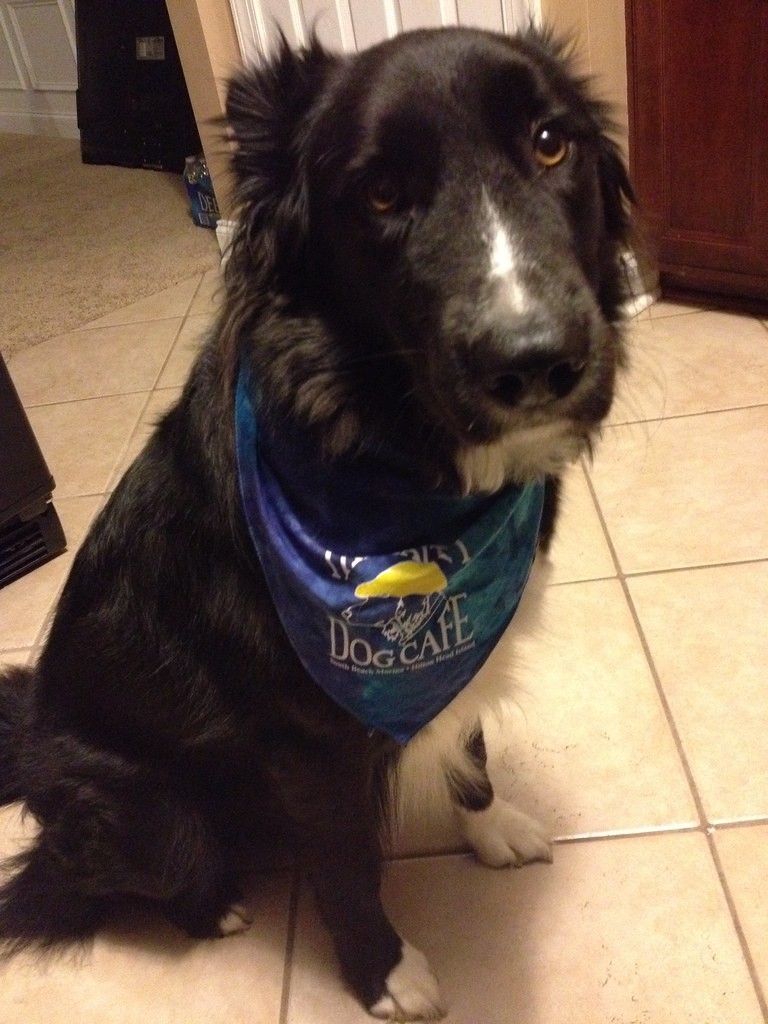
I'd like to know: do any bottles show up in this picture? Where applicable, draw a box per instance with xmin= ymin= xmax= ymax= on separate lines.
xmin=183 ymin=154 xmax=203 ymax=185
xmin=198 ymin=158 xmax=214 ymax=193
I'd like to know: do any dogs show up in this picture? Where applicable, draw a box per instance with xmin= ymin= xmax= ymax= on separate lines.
xmin=1 ymin=10 xmax=642 ymax=1024
